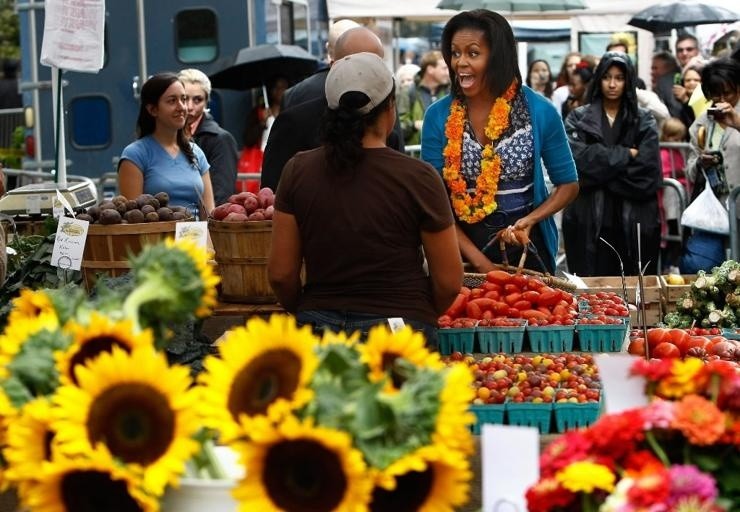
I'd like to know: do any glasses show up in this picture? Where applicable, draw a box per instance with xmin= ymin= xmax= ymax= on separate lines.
xmin=678 ymin=47 xmax=697 ymax=51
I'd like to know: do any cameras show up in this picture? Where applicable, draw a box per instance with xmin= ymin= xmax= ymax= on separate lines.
xmin=707 ymin=109 xmax=725 ymax=122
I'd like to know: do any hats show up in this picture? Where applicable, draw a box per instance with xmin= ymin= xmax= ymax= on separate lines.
xmin=323 ymin=52 xmax=392 ymax=113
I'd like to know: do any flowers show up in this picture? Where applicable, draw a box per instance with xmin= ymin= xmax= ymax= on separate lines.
xmin=2 ymin=237 xmax=475 ymax=512
xmin=527 ymin=356 xmax=734 ymax=510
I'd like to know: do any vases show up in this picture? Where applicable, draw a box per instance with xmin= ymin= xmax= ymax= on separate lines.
xmin=169 ymin=443 xmax=257 ymax=511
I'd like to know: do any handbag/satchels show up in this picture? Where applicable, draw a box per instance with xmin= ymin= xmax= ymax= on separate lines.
xmin=462 ymin=226 xmax=576 ymax=292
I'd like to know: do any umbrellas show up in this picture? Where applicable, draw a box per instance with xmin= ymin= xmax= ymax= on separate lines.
xmin=205 ymin=45 xmax=319 ymax=91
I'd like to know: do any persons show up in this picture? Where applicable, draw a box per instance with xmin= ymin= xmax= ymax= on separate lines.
xmin=650 ymin=53 xmax=678 ymax=92
xmin=660 ymin=118 xmax=694 ymax=275
xmin=603 ymin=44 xmax=670 ymax=142
xmin=656 ymin=37 xmax=698 ymax=114
xmin=392 ymin=63 xmax=423 ymax=102
xmin=566 ymin=52 xmax=660 ymax=279
xmin=417 ymin=11 xmax=581 ymax=278
xmin=398 ymin=50 xmax=459 ymax=161
xmin=683 ymin=61 xmax=740 ymax=267
xmin=262 ymin=26 xmax=407 ymax=190
xmin=627 ymin=2 xmax=739 ymax=33
xmin=688 ymin=25 xmax=740 ymax=119
xmin=260 ymin=76 xmax=290 ymax=133
xmin=268 ymin=53 xmax=464 ymax=345
xmin=549 ymin=54 xmax=583 ymax=114
xmin=173 ymin=69 xmax=238 ymax=206
xmin=527 ymin=60 xmax=556 ymax=109
xmin=557 ymin=58 xmax=600 ymax=121
xmin=673 ymin=56 xmax=705 ymax=143
xmin=119 ymin=72 xmax=214 ymax=220
